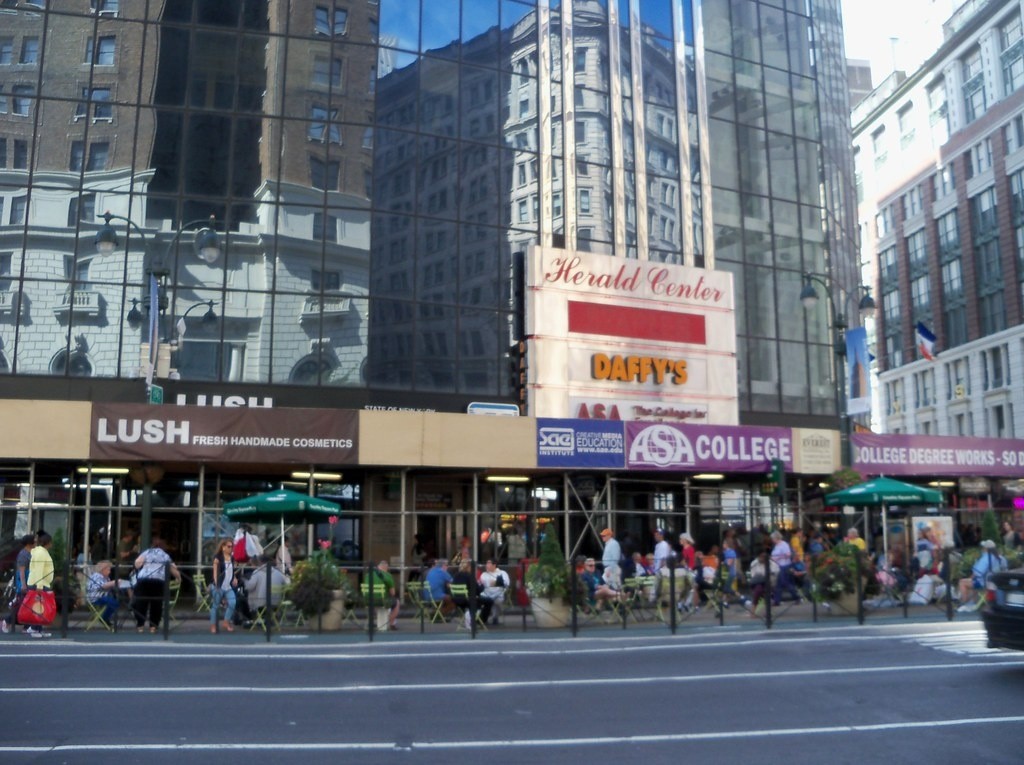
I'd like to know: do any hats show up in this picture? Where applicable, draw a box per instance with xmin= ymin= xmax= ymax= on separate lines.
xmin=981 ymin=540 xmax=995 ymax=548
xmin=600 ymin=528 xmax=613 ymax=535
xmin=680 ymin=532 xmax=694 ymax=544
xmin=124 ymin=529 xmax=137 ymax=535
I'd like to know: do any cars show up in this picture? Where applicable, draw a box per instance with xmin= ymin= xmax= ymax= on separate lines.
xmin=982 ymin=565 xmax=1024 ymax=653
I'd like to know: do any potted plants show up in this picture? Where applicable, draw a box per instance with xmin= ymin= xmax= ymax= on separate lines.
xmin=525 ymin=522 xmax=571 ymax=627
xmin=50 ymin=525 xmax=77 ymax=614
xmin=760 ymin=456 xmax=785 ymax=497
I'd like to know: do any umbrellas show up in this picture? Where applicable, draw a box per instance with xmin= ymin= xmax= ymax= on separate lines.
xmin=826 ymin=476 xmax=944 ymax=571
xmin=224 ymin=490 xmax=340 ymax=575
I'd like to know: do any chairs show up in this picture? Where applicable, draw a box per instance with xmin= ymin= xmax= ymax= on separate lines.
xmin=84 ymin=576 xmax=305 ymax=634
xmin=406 ymin=582 xmax=447 ymax=625
xmin=449 ymin=584 xmax=489 ymax=632
xmin=580 ymin=572 xmax=763 ymax=625
xmin=362 ymin=583 xmax=386 ymax=629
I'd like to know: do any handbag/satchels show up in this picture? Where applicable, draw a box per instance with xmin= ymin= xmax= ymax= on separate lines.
xmin=233 ymin=528 xmax=264 ymax=562
xmin=206 ymin=583 xmax=213 ymax=593
xmin=18 ymin=590 xmax=57 ymax=624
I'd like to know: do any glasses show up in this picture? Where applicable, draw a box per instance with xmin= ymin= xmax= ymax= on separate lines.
xmin=226 ymin=545 xmax=233 ymax=548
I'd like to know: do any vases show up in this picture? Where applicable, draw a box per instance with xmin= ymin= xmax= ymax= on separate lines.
xmin=308 ymin=591 xmax=345 ymax=630
xmin=829 ymin=589 xmax=858 ymax=616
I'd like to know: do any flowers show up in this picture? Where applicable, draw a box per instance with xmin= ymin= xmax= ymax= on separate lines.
xmin=818 ymin=465 xmax=865 ymax=493
xmin=308 ymin=516 xmax=346 ymax=590
xmin=809 ymin=543 xmax=879 ymax=602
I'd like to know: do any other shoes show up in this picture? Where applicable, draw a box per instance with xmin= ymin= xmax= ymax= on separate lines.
xmin=2 ymin=620 xmax=11 ymax=633
xmin=210 ymin=624 xmax=215 ymax=633
xmin=40 ymin=631 xmax=52 ymax=638
xmin=957 ymin=605 xmax=972 ymax=612
xmin=27 ymin=626 xmax=42 ymax=638
xmin=223 ymin=620 xmax=234 ymax=632
xmin=150 ymin=627 xmax=158 ymax=634
xmin=22 ymin=627 xmax=27 ymax=633
xmin=493 ymin=616 xmax=498 ymax=624
xmin=136 ymin=626 xmax=143 ymax=633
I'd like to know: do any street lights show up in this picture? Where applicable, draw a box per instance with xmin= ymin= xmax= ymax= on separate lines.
xmin=803 ymin=269 xmax=879 ymax=589
xmin=93 ymin=204 xmax=224 ymax=551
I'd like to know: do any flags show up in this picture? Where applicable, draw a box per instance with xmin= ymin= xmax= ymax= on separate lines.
xmin=915 ymin=320 xmax=937 ymax=362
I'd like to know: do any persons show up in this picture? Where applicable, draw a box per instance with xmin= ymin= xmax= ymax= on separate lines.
xmin=128 ymin=462 xmax=185 ymax=507
xmin=423 ymin=558 xmax=511 ymax=628
xmin=77 ymin=528 xmax=179 ymax=634
xmin=575 ymin=525 xmax=882 ymax=616
xmin=913 ymin=522 xmax=1023 ymax=603
xmin=363 ymin=560 xmax=400 ymax=631
xmin=2 ymin=531 xmax=55 ymax=639
xmin=460 ymin=536 xmax=472 ymax=559
xmin=209 ymin=534 xmax=303 ymax=634
xmin=405 ymin=534 xmax=426 ymax=592
